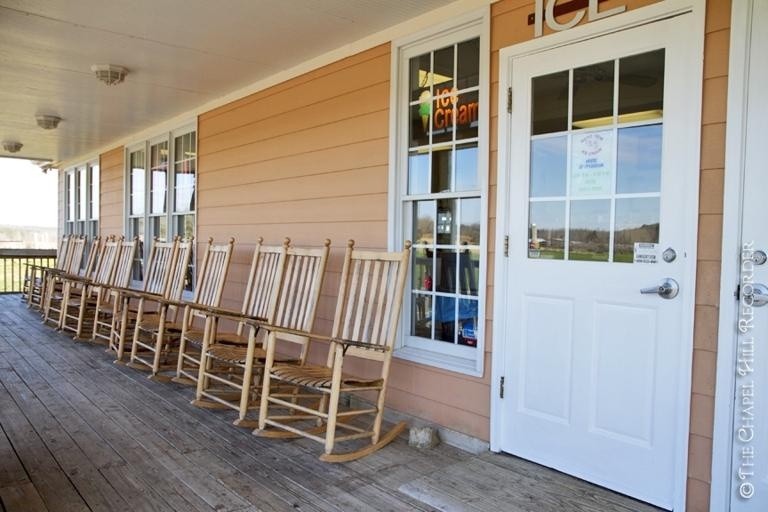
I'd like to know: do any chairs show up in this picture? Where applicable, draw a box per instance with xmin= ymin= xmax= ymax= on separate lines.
xmin=253 ymin=240 xmax=410 ymax=462
xmin=124 ymin=237 xmax=238 ymax=382
xmin=189 ymin=239 xmax=333 ymax=428
xmin=73 ymin=235 xmax=140 ymax=349
xmin=172 ymin=237 xmax=290 ymax=403
xmin=105 ymin=235 xmax=195 ymax=368
xmin=20 ymin=235 xmax=100 ymax=337
xmin=424 ymin=241 xmax=477 ymax=342
